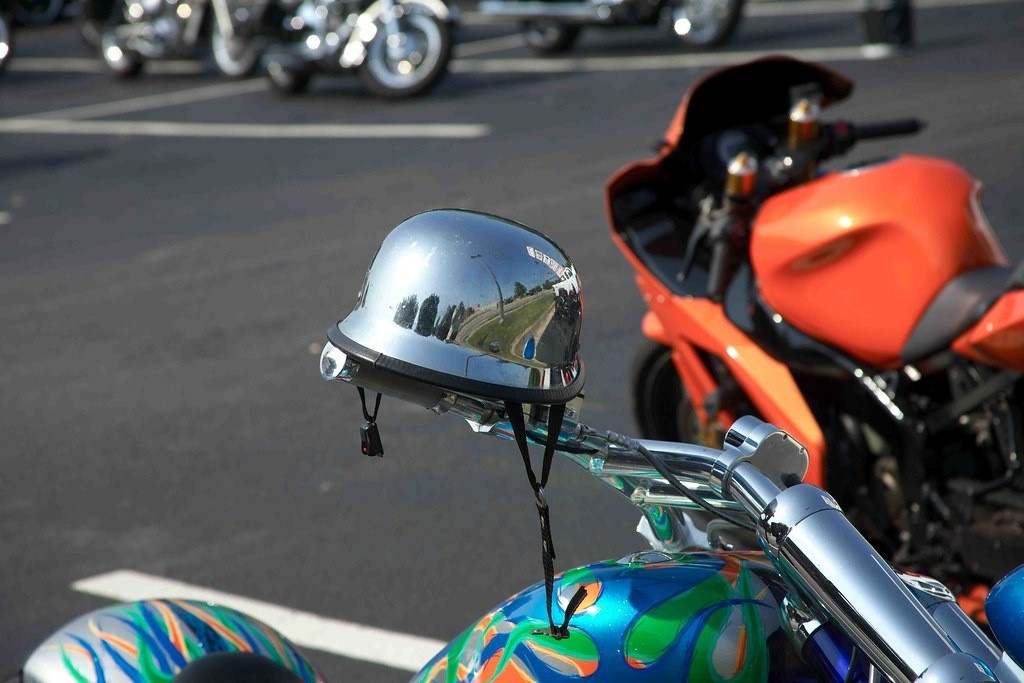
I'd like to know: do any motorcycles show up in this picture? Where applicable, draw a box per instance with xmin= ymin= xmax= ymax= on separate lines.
xmin=18 ymin=337 xmax=1024 ymax=681
xmin=603 ymin=51 xmax=1023 ymax=629
xmin=0 ymin=0 xmax=920 ymax=103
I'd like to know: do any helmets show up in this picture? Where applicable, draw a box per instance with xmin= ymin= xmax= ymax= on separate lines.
xmin=327 ymin=208 xmax=586 ymax=404
